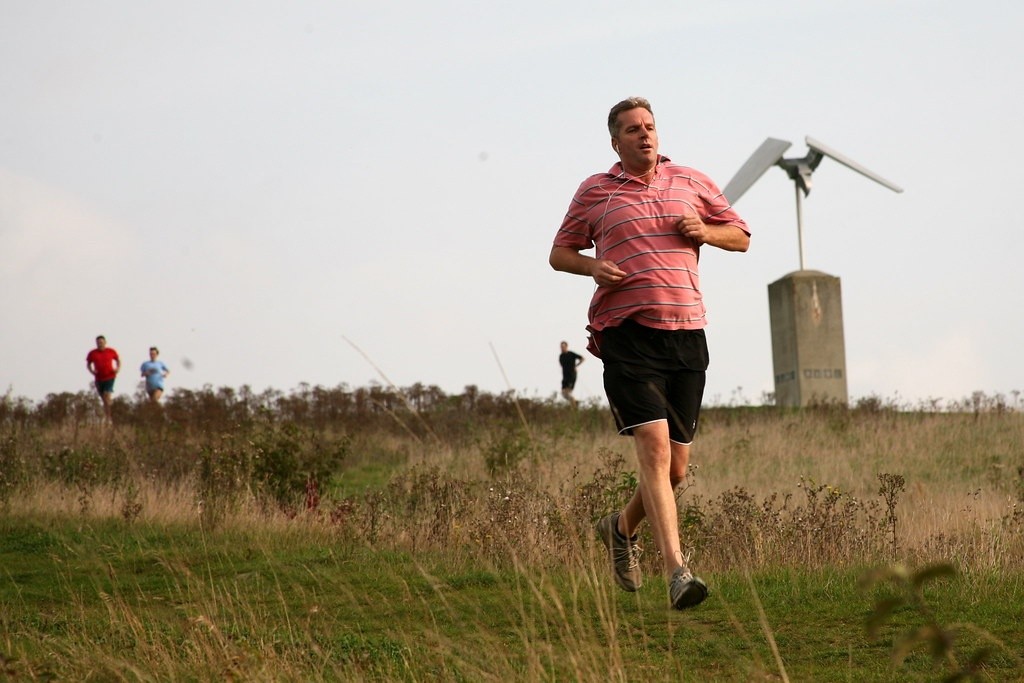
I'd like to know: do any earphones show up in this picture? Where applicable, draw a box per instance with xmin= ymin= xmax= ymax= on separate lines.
xmin=616 ymin=145 xmax=620 ymax=153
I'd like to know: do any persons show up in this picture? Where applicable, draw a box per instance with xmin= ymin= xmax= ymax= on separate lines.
xmin=558 ymin=342 xmax=585 ymax=410
xmin=548 ymin=97 xmax=753 ymax=611
xmin=140 ymin=346 xmax=170 ymax=408
xmin=85 ymin=335 xmax=121 ymax=426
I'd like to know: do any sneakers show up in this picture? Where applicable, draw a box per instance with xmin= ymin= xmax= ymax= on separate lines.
xmin=669 ymin=547 xmax=708 ymax=610
xmin=597 ymin=513 xmax=644 ymax=592
